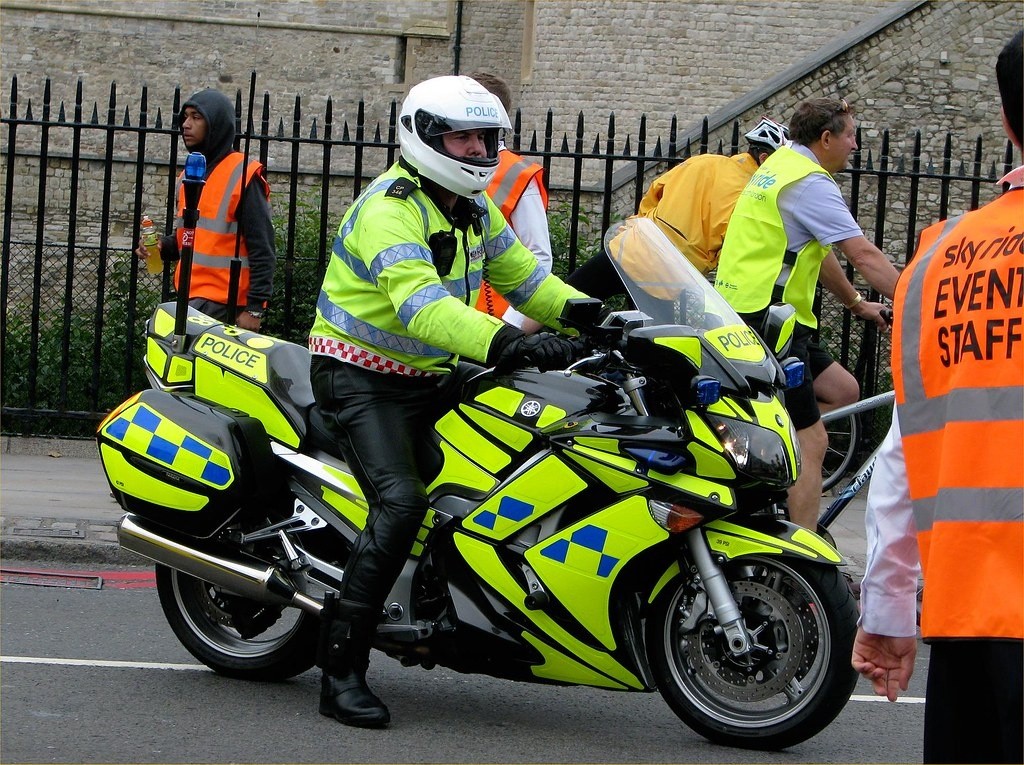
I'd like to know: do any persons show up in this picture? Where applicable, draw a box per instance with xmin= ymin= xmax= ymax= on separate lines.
xmin=136 ymin=88 xmax=277 ymax=335
xmin=309 ymin=71 xmax=612 ymax=726
xmin=564 ymin=99 xmax=901 ymax=533
xmin=850 ymin=28 xmax=1024 ymax=765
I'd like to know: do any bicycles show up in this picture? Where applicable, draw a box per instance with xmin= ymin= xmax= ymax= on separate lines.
xmin=786 ymin=307 xmax=899 ymax=553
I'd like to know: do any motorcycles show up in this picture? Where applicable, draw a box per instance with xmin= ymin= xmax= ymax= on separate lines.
xmin=92 ymin=152 xmax=864 ymax=752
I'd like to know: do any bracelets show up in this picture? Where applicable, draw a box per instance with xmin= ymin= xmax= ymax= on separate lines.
xmin=843 ymin=292 xmax=862 ymax=309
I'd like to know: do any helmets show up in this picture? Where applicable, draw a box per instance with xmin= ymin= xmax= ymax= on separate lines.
xmin=398 ymin=75 xmax=512 ymax=198
xmin=745 ymin=117 xmax=794 ymax=153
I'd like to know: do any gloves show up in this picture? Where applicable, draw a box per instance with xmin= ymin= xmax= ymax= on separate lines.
xmin=494 ymin=331 xmax=576 ymax=375
xmin=590 ymin=306 xmax=641 ymax=348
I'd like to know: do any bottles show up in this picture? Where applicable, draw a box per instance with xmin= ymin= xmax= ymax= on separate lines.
xmin=142 ymin=216 xmax=163 ymax=276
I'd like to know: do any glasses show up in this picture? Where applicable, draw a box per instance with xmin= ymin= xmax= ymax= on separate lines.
xmin=831 ymin=99 xmax=849 ymax=116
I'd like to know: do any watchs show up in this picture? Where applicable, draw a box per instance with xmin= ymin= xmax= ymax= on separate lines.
xmin=247 ymin=311 xmax=265 ymax=318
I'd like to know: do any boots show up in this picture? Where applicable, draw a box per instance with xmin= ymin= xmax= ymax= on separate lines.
xmin=318 ymin=591 xmax=389 ymax=727
